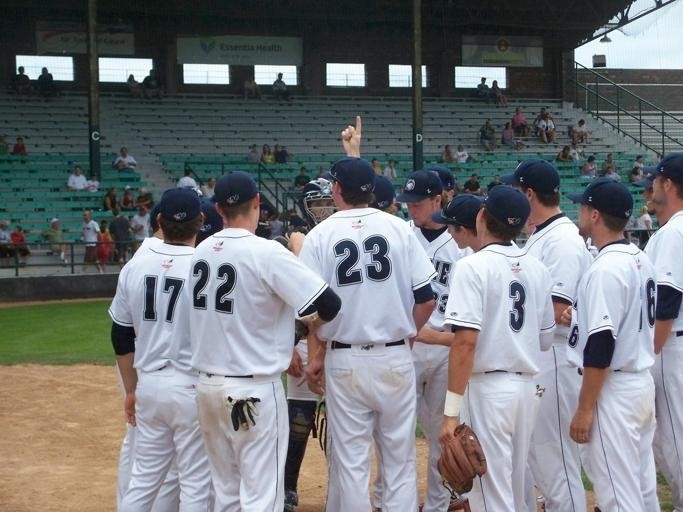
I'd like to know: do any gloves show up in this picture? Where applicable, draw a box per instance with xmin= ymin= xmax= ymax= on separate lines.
xmin=226 ymin=395 xmax=264 ymax=433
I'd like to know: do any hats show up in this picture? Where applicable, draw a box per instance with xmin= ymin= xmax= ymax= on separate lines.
xmin=632 ymin=151 xmax=682 ymax=189
xmin=49 ymin=217 xmax=62 ymax=226
xmin=319 ymin=155 xmax=377 ymax=192
xmin=568 ymin=175 xmax=635 ymax=218
xmin=431 ymin=193 xmax=484 ymax=226
xmin=214 ymin=170 xmax=264 ymax=207
xmin=365 ymin=163 xmax=457 ymax=209
xmin=484 ymin=183 xmax=533 ymax=229
xmin=147 ymin=186 xmax=224 ymax=243
xmin=498 ymin=158 xmax=562 ymax=193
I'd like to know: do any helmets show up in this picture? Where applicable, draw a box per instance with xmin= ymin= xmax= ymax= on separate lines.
xmin=299 ymin=176 xmax=338 ymax=228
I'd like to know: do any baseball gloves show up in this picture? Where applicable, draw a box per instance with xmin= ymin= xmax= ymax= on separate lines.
xmin=438 ymin=424 xmax=487 ymax=496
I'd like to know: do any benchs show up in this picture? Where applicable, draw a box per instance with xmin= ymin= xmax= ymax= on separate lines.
xmin=1 ymin=75 xmax=681 ymax=280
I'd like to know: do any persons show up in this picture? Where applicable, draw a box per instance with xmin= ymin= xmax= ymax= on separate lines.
xmin=243 ymin=75 xmax=266 ymax=100
xmin=68 ymin=166 xmax=154 ymax=274
xmin=476 ymin=76 xmax=589 ymax=153
xmin=14 ymin=68 xmax=59 ymax=99
xmin=1 ymin=221 xmax=31 ymax=267
xmin=127 ymin=72 xmax=164 ymax=99
xmin=13 ymin=138 xmax=27 ymax=165
xmin=272 ymin=74 xmax=289 ymax=101
xmin=41 ymin=219 xmax=65 ymax=258
xmin=106 ymin=115 xmax=683 ymax=511
